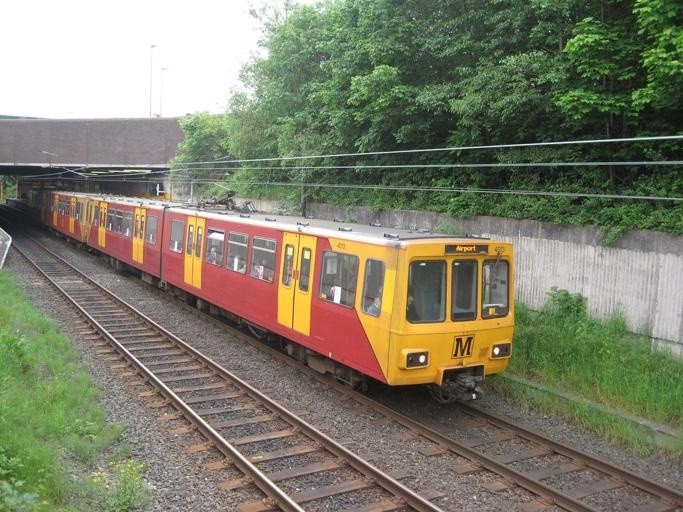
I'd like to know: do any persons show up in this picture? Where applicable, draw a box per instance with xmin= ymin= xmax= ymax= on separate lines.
xmin=230 ymin=256 xmax=247 ymax=274
xmin=205 ymin=247 xmax=221 ymax=263
xmin=253 ymin=257 xmax=272 ymax=280
xmin=365 ymin=294 xmax=382 ymax=316
xmin=405 ymin=295 xmax=417 ymax=318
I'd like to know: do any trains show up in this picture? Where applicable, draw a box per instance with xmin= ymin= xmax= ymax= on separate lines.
xmin=28 ymin=187 xmax=513 ymax=404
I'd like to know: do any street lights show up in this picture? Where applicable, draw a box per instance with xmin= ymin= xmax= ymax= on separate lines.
xmin=158 ymin=66 xmax=169 ymax=117
xmin=148 ymin=43 xmax=158 ymax=118
